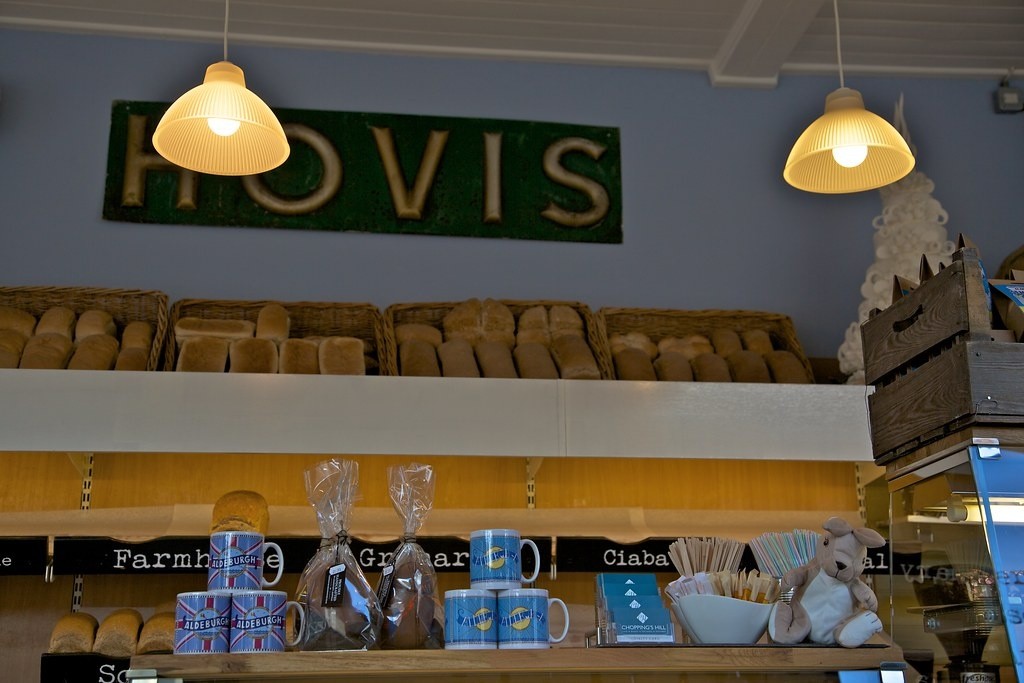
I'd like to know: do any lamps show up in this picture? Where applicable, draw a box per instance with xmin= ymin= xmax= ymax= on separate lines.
xmin=782 ymin=0 xmax=916 ymax=195
xmin=148 ymin=0 xmax=290 ymax=177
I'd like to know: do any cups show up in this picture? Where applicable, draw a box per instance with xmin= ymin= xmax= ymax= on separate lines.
xmin=776 ymin=575 xmax=800 ymax=606
xmin=972 ymin=596 xmax=1003 ymax=637
xmin=207 ymin=530 xmax=284 ymax=591
xmin=230 ymin=591 xmax=306 ymax=653
xmin=498 ymin=589 xmax=570 ymax=649
xmin=172 ymin=591 xmax=231 ymax=654
xmin=469 ymin=529 xmax=541 ymax=590
xmin=444 ymin=590 xmax=498 ymax=650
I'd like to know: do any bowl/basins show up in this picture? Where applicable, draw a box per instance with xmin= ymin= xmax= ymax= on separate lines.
xmin=670 ymin=594 xmax=774 ymax=646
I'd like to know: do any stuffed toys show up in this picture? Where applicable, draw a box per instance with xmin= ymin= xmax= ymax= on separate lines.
xmin=767 ymin=516 xmax=887 ymax=650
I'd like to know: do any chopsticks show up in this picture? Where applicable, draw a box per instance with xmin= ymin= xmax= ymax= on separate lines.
xmin=667 ymin=535 xmax=746 ymax=578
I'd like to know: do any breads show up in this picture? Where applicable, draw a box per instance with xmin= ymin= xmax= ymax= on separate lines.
xmin=607 ymin=326 xmax=811 ymax=384
xmin=292 ymin=555 xmax=444 ymax=650
xmin=210 ymin=490 xmax=268 ymax=536
xmin=0 ymin=304 xmax=150 ymax=369
xmin=172 ymin=304 xmax=372 ymax=376
xmin=47 ymin=608 xmax=175 ymax=657
xmin=393 ymin=296 xmax=600 ymax=380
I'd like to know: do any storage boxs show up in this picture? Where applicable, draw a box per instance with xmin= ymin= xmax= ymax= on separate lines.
xmin=860 ymin=253 xmax=1023 ymax=467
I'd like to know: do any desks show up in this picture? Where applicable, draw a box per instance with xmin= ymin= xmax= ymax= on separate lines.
xmin=129 ymin=644 xmax=904 ymax=683
